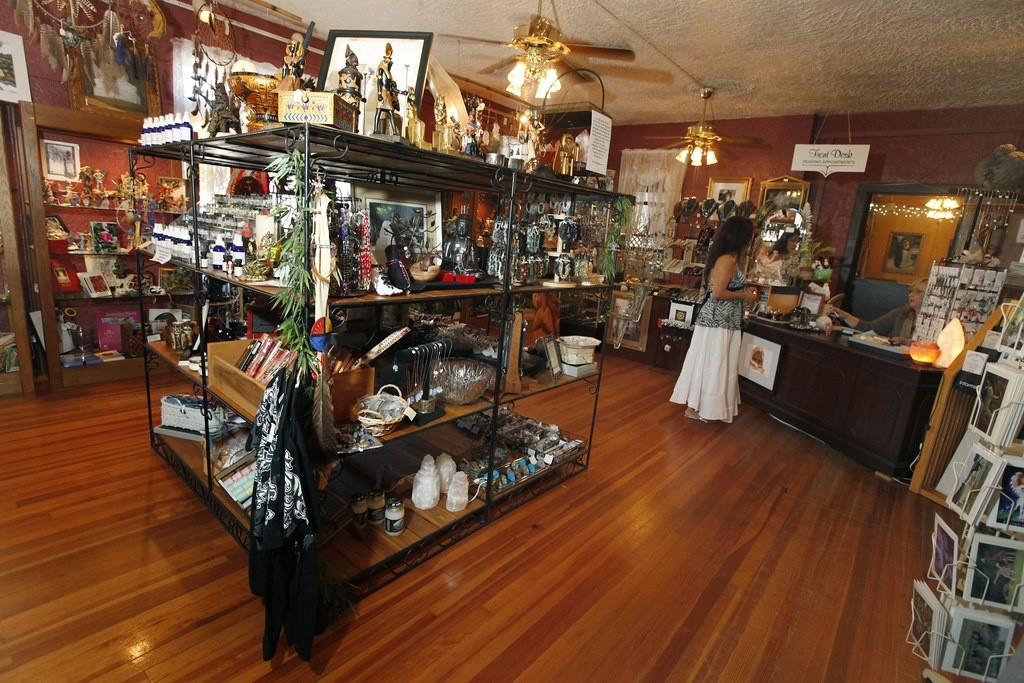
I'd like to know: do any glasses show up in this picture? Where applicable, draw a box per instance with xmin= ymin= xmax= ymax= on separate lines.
xmin=908 ymin=287 xmax=924 ymax=294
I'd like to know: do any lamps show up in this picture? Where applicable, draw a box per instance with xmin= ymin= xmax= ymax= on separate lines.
xmin=505 ymin=60 xmax=561 ymax=99
xmin=675 ymin=145 xmax=718 ymax=166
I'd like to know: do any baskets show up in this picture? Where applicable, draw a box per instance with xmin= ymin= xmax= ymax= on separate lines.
xmin=161 ymin=393 xmax=224 ymax=436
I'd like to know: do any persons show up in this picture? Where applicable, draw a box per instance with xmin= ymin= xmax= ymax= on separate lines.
xmin=442 ymin=213 xmax=486 ymax=281
xmin=830 ymin=276 xmax=929 ymax=347
xmin=336 ymin=44 xmax=367 ymax=109
xmin=668 ymin=217 xmax=761 ymax=424
xmin=374 ymin=42 xmax=408 ymax=136
xmin=281 ymin=37 xmax=305 ymax=80
xmin=770 ymin=229 xmax=801 ymax=262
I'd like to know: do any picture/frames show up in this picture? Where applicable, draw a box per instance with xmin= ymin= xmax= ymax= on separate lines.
xmin=737 ymin=331 xmax=786 ymax=396
xmin=365 ymin=199 xmax=429 ymax=255
xmin=760 ymin=176 xmax=810 ymax=229
xmin=882 ymin=230 xmax=924 ymax=275
xmin=606 ymin=289 xmax=653 ymax=352
xmin=668 ymin=300 xmax=694 ymax=331
xmin=64 ymin=35 xmax=163 ymax=119
xmin=707 ymin=177 xmax=751 ymax=221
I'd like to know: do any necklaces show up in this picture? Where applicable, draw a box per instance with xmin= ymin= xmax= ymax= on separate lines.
xmin=739 ymin=204 xmax=752 ymax=217
xmin=701 ymin=202 xmax=715 ymax=218
xmin=719 ymin=203 xmax=737 ymax=222
xmin=336 ymin=209 xmax=372 ymax=297
xmin=698 ymin=229 xmax=709 ymax=244
xmin=684 ymin=200 xmax=697 ymax=221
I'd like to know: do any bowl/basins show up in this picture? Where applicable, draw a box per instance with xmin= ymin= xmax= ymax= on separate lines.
xmin=556 ymin=335 xmax=602 ymax=365
xmin=909 ymin=345 xmax=941 ymax=367
xmin=430 ymin=356 xmax=494 ymax=406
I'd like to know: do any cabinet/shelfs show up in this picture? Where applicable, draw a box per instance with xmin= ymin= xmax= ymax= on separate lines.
xmin=43 ymin=202 xmax=219 ymax=391
xmin=767 ymin=329 xmax=923 ymax=476
xmin=129 ymin=121 xmax=635 ymax=611
xmin=653 ymin=297 xmax=703 ymax=370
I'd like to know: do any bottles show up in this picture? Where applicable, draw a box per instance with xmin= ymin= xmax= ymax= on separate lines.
xmin=349 ymin=492 xmax=367 ymax=528
xmin=170 ymin=322 xmax=187 ymax=354
xmin=181 ymin=319 xmax=195 ymax=349
xmin=367 ymin=488 xmax=385 ymax=525
xmin=140 ymin=112 xmax=191 ymax=146
xmin=384 ymin=498 xmax=405 ymax=537
xmin=151 ymin=223 xmax=246 ymax=278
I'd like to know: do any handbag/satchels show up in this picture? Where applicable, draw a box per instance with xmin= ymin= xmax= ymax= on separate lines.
xmin=813 ymin=265 xmax=830 ymax=280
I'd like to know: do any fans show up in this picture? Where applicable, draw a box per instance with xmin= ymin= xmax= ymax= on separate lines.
xmin=438 ymin=0 xmax=637 ymax=79
xmin=644 ymin=86 xmax=758 ymax=147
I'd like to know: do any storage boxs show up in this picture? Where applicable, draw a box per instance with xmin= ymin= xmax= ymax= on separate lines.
xmin=562 ymin=362 xmax=598 ymax=377
xmin=206 ymin=339 xmax=373 ymax=428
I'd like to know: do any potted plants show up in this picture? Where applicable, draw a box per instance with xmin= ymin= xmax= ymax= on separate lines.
xmin=791 ymin=238 xmax=837 ymax=282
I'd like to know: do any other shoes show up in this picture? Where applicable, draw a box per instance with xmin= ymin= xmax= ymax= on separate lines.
xmin=684 ymin=407 xmax=699 ymax=420
xmin=697 ymin=414 xmax=722 ymax=424
